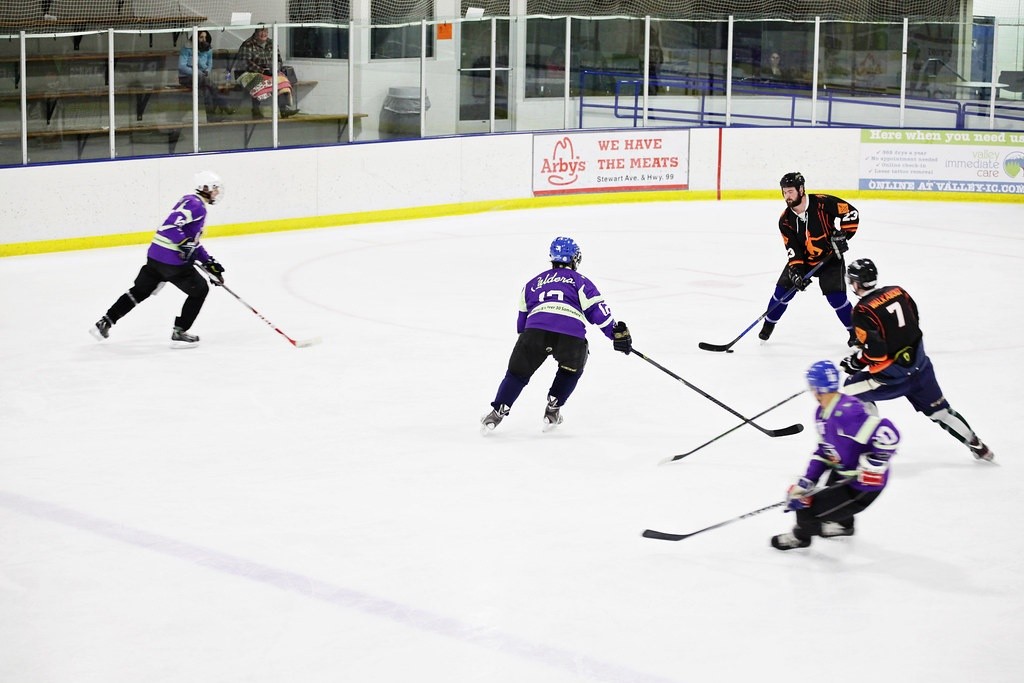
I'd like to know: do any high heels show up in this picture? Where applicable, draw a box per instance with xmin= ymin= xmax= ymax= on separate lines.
xmin=206 ymin=113 xmax=225 ymax=124
xmin=218 ymin=103 xmax=237 ymax=114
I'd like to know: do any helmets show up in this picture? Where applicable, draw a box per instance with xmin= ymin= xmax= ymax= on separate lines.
xmin=548 ymin=236 xmax=582 ymax=272
xmin=805 ymin=359 xmax=839 ymax=394
xmin=193 ymin=170 xmax=221 ymax=205
xmin=779 ymin=172 xmax=804 ymax=196
xmin=846 ymin=258 xmax=877 ymax=290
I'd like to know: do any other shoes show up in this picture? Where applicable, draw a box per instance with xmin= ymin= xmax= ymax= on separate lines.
xmin=279 ymin=104 xmax=300 ymax=119
xmin=252 ymin=110 xmax=264 ymax=120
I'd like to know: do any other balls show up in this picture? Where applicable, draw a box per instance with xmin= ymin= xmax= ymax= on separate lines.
xmin=726 ymin=349 xmax=734 ymax=353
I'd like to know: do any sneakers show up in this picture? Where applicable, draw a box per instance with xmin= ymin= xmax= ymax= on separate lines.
xmin=480 ymin=403 xmax=510 ymax=436
xmin=758 ymin=318 xmax=776 ymax=345
xmin=818 ymin=520 xmax=855 ymax=538
xmin=770 ymin=533 xmax=812 ymax=551
xmin=543 ymin=395 xmax=564 ymax=433
xmin=90 ymin=315 xmax=113 ymax=343
xmin=970 ymin=437 xmax=994 ymax=461
xmin=847 ymin=328 xmax=859 ymax=351
xmin=171 ymin=316 xmax=200 ymax=350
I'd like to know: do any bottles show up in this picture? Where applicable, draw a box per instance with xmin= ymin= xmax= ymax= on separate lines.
xmin=326 ymin=49 xmax=333 ymax=59
xmin=224 ymin=67 xmax=231 ymax=87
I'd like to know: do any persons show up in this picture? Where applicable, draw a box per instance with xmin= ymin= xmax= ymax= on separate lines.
xmin=840 ymin=258 xmax=994 ymax=462
xmin=764 ymin=52 xmax=791 ymax=82
xmin=234 ymin=22 xmax=300 ymax=120
xmin=770 ymin=360 xmax=900 ymax=550
xmin=758 ymin=172 xmax=859 ymax=348
xmin=480 ymin=236 xmax=632 ymax=435
xmin=178 ymin=30 xmax=238 ymax=123
xmin=89 ymin=171 xmax=225 ymax=349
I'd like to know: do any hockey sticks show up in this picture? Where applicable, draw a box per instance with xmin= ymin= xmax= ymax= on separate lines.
xmin=629 ymin=346 xmax=804 ymax=438
xmin=642 ymin=499 xmax=791 ymax=542
xmin=192 ymin=261 xmax=323 ymax=348
xmin=698 ymin=257 xmax=823 ymax=352
xmin=659 ymin=367 xmax=846 ymax=466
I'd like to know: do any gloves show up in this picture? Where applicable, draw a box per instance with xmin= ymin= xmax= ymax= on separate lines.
xmin=179 ymin=236 xmax=199 ymax=261
xmin=789 ymin=262 xmax=812 ymax=291
xmin=613 ymin=321 xmax=632 ymax=355
xmin=830 ymin=230 xmax=849 ymax=258
xmin=784 ymin=477 xmax=814 ymax=513
xmin=857 ymin=452 xmax=887 ymax=486
xmin=202 ymin=256 xmax=226 ymax=286
xmin=839 ymin=351 xmax=867 ymax=376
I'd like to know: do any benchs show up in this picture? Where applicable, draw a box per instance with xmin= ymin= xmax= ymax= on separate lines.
xmin=0 ymin=16 xmax=368 ymax=161
